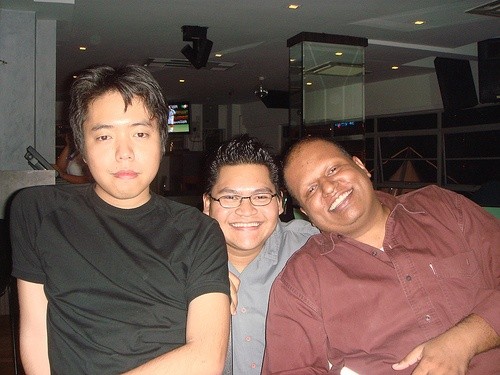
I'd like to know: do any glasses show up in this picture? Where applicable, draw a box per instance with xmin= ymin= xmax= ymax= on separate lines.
xmin=209 ymin=193 xmax=278 ymax=208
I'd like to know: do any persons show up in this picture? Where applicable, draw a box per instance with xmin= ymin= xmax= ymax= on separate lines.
xmin=203 ymin=138 xmax=321 ymax=375
xmin=52 ymin=132 xmax=96 ymax=184
xmin=261 ymin=138 xmax=500 ymax=375
xmin=10 ymin=64 xmax=232 ymax=375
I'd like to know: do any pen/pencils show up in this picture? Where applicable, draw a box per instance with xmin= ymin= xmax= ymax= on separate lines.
xmin=430 ymin=263 xmax=436 ymax=275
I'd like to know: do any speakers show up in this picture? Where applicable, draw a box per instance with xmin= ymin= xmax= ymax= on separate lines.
xmin=434 ymin=56 xmax=478 ymax=111
xmin=477 ymin=37 xmax=500 ymax=104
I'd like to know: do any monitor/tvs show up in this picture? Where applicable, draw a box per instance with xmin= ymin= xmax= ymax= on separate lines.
xmin=167 ymin=102 xmax=192 ymax=135
xmin=24 ymin=146 xmax=59 ymax=178
